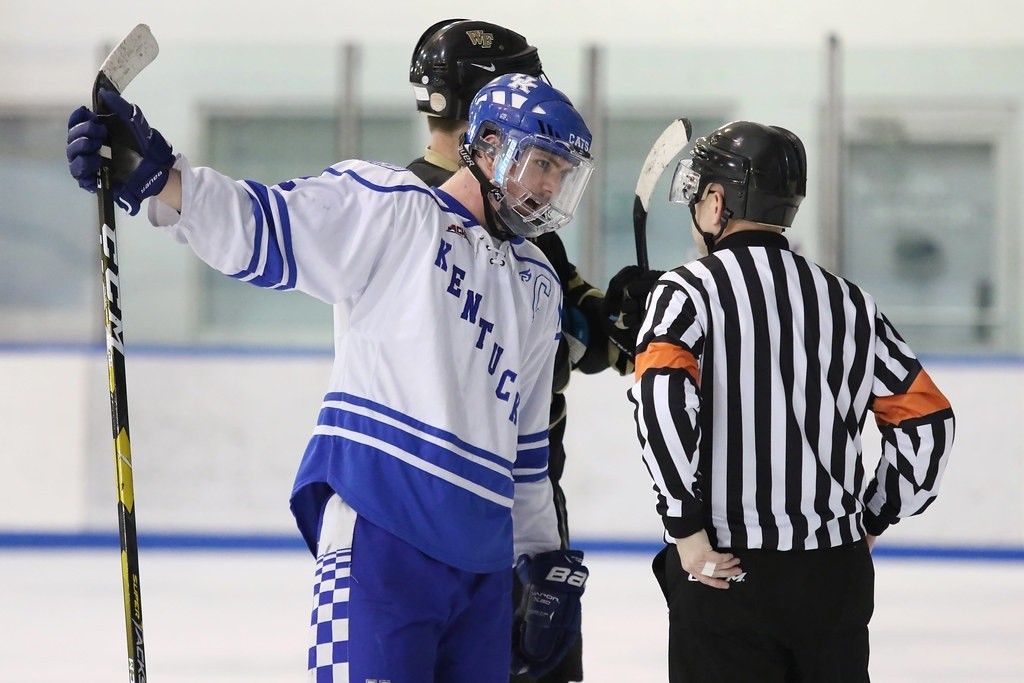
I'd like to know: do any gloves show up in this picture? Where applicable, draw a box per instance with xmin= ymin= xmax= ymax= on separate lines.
xmin=67 ymin=87 xmax=176 ymax=216
xmin=510 ymin=549 xmax=589 ymax=679
xmin=603 ymin=264 xmax=667 ymax=377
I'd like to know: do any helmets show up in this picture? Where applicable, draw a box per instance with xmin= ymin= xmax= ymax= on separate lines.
xmin=464 ymin=73 xmax=595 ymax=237
xmin=409 ymin=19 xmax=553 ymax=121
xmin=669 ymin=121 xmax=807 ymax=227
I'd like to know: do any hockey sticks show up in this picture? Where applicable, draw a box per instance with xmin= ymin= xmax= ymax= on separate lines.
xmin=84 ymin=18 xmax=165 ymax=683
xmin=627 ymin=113 xmax=696 ymax=273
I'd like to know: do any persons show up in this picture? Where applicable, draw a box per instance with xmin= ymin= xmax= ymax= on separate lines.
xmin=405 ymin=15 xmax=671 ymax=683
xmin=628 ymin=117 xmax=957 ymax=683
xmin=67 ymin=73 xmax=589 ymax=683
